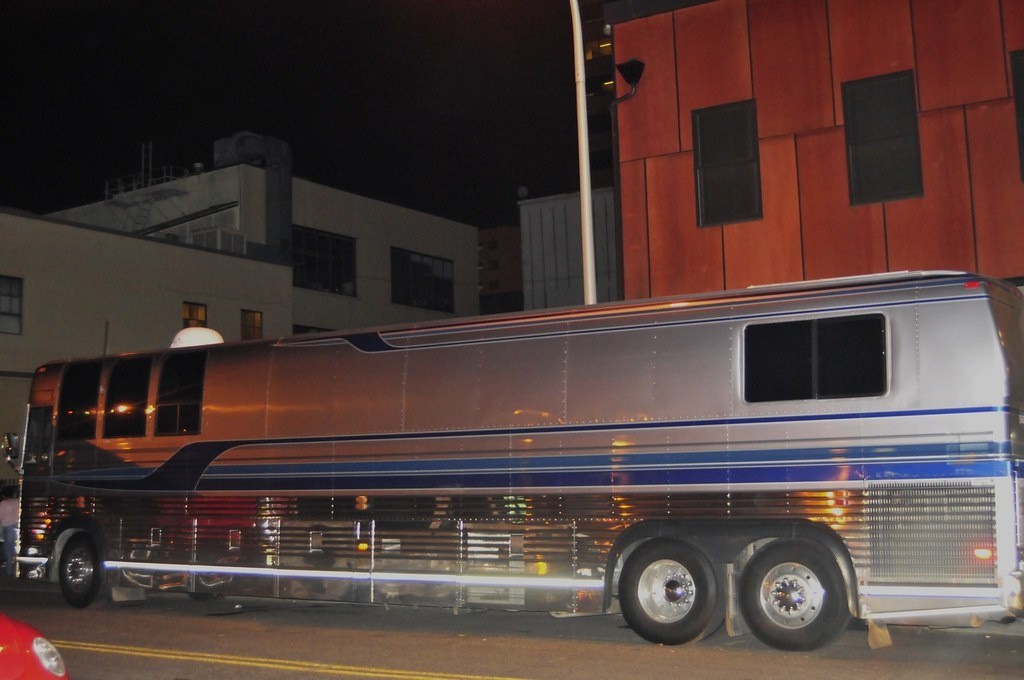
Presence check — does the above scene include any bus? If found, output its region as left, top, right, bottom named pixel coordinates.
left=12, top=264, right=1024, bottom=644
